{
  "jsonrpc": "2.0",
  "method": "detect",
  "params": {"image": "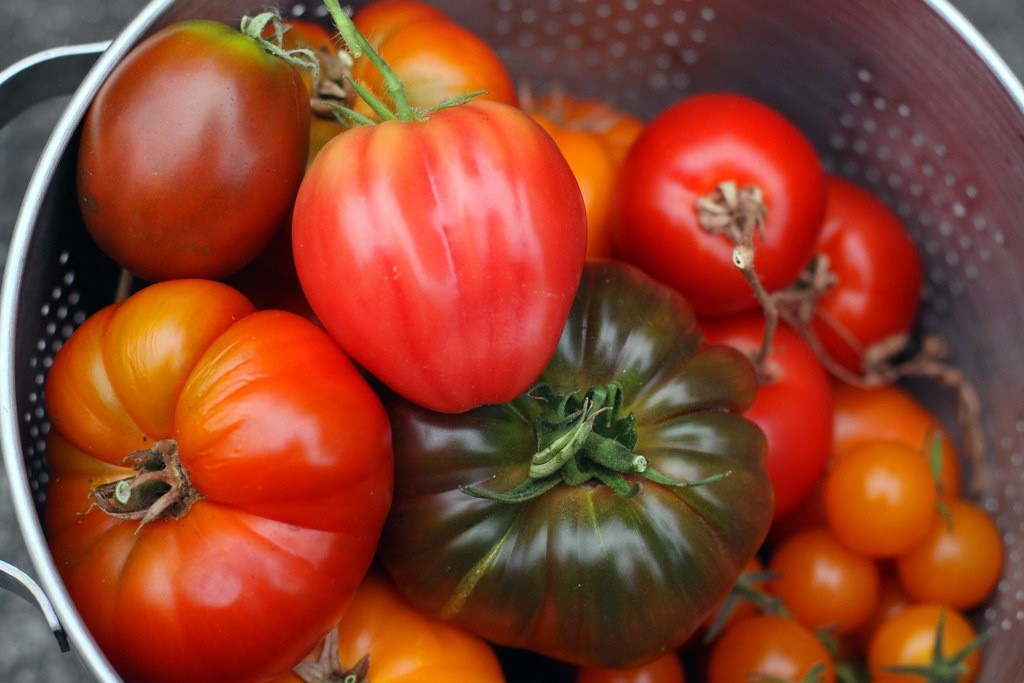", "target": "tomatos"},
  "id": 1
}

[40,2,1007,683]
